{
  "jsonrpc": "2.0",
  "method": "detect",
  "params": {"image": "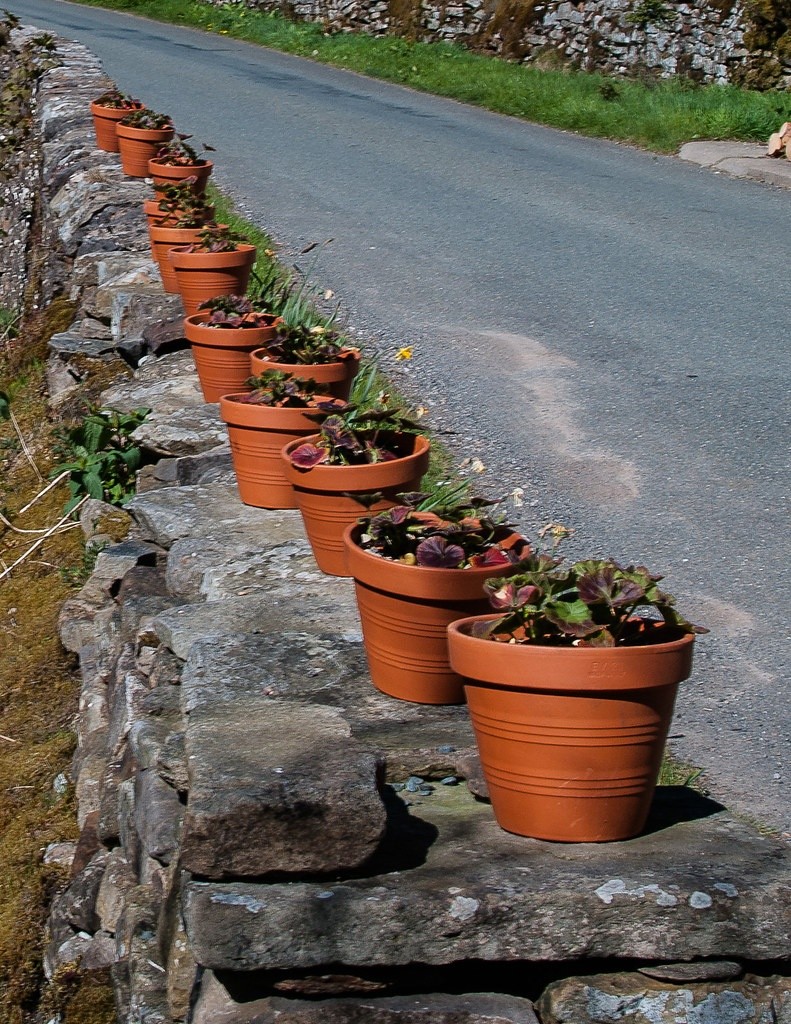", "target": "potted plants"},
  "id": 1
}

[343,489,531,706]
[282,390,433,577]
[116,107,177,178]
[90,83,146,151]
[447,556,696,844]
[167,226,257,316]
[145,174,217,260]
[150,198,230,295]
[148,133,213,200]
[249,320,360,403]
[219,368,347,508]
[185,294,286,403]
